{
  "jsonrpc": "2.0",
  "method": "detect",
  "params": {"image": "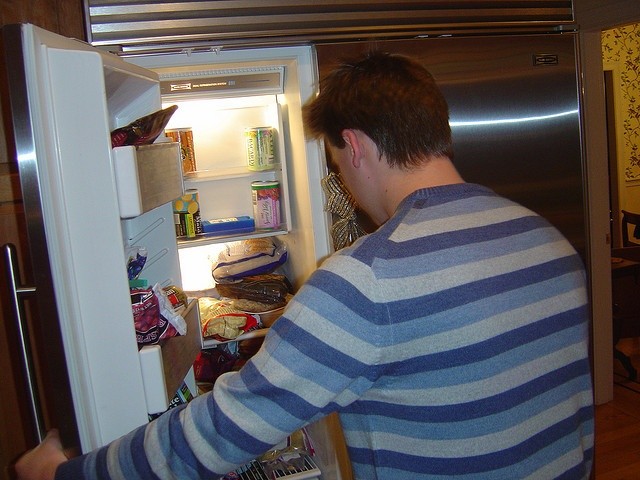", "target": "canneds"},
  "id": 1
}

[245,125,275,171]
[165,127,197,179]
[172,189,203,239]
[251,180,281,232]
